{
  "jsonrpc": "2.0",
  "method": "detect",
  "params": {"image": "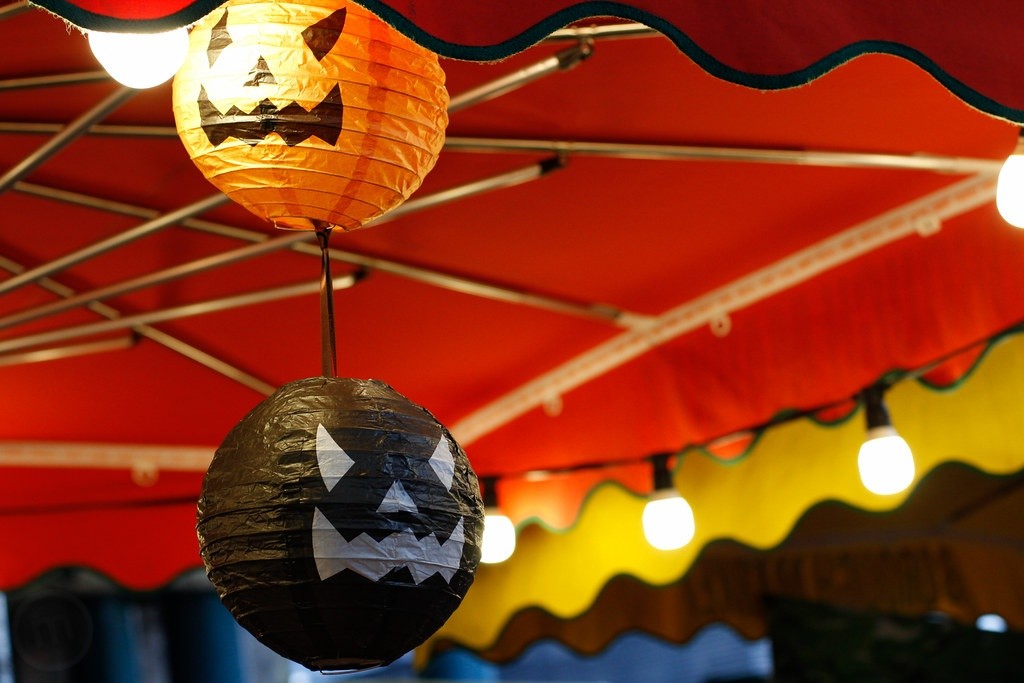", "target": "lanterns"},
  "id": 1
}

[172,0,453,234]
[197,375,483,671]
